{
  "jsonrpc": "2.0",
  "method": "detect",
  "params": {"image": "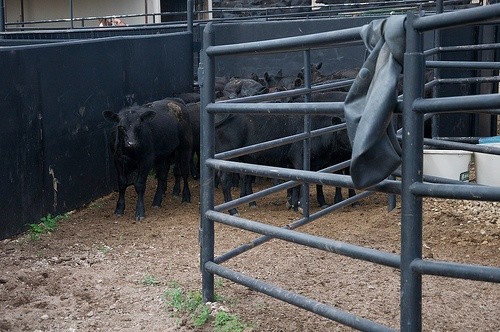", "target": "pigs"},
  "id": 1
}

[100,61,361,220]
